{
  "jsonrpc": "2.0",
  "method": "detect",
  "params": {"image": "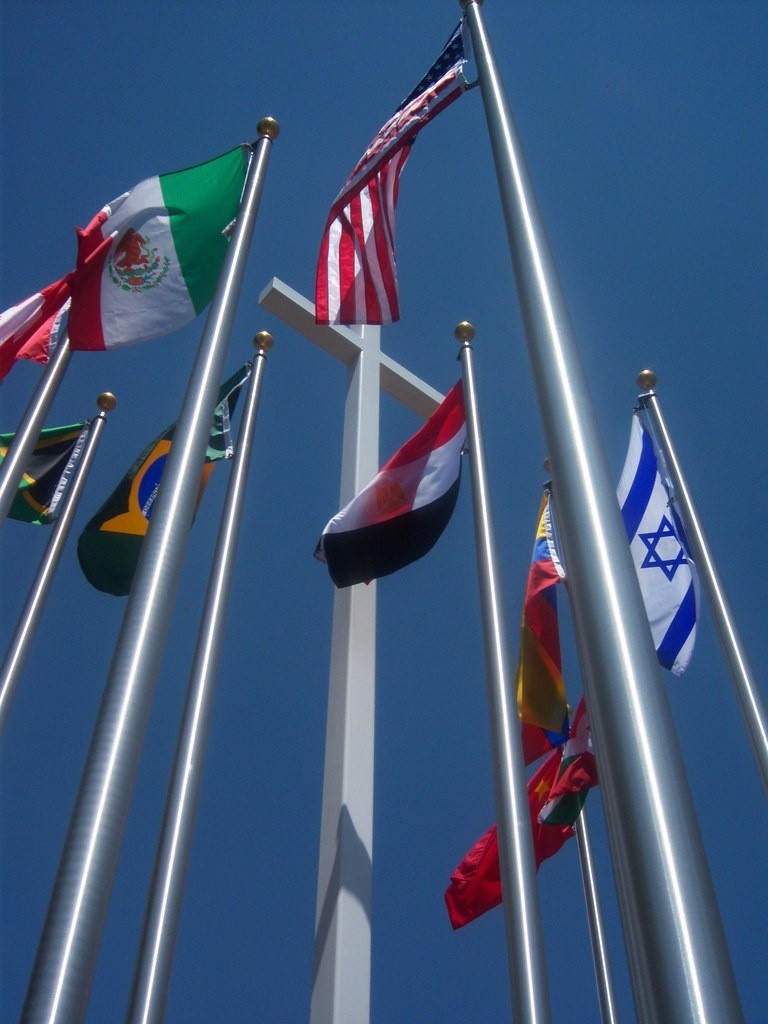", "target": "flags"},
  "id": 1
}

[445,695,598,935]
[2,274,83,382]
[2,422,96,526]
[65,134,256,355]
[74,357,254,598]
[614,407,706,677]
[510,490,571,767]
[317,370,468,589]
[299,13,477,330]
